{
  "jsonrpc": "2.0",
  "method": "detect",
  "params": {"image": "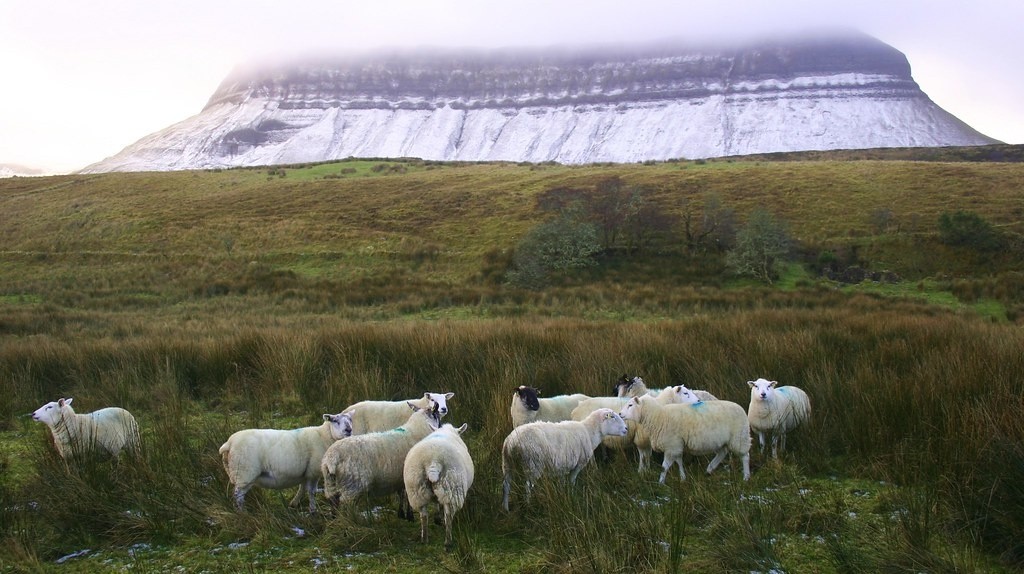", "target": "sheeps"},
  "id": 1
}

[321,401,441,520]
[217,409,357,514]
[32,397,140,466]
[340,392,456,432]
[403,423,475,547]
[502,408,629,514]
[747,378,812,459]
[510,386,590,429]
[571,372,751,485]
